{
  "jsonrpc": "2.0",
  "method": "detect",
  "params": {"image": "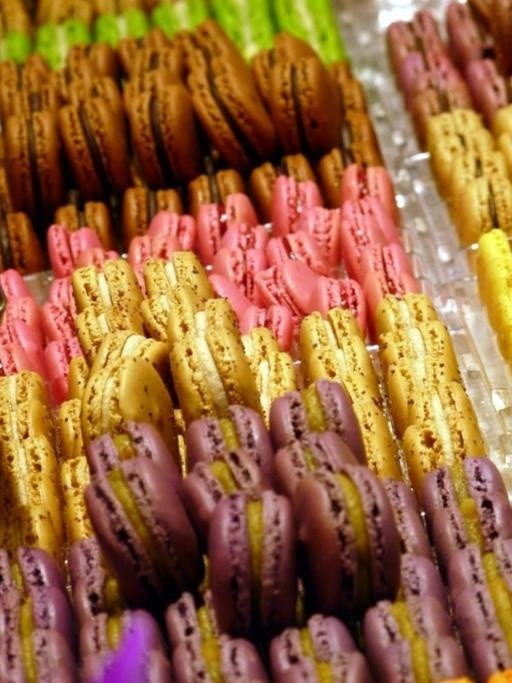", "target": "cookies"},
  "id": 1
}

[0,0,512,683]
